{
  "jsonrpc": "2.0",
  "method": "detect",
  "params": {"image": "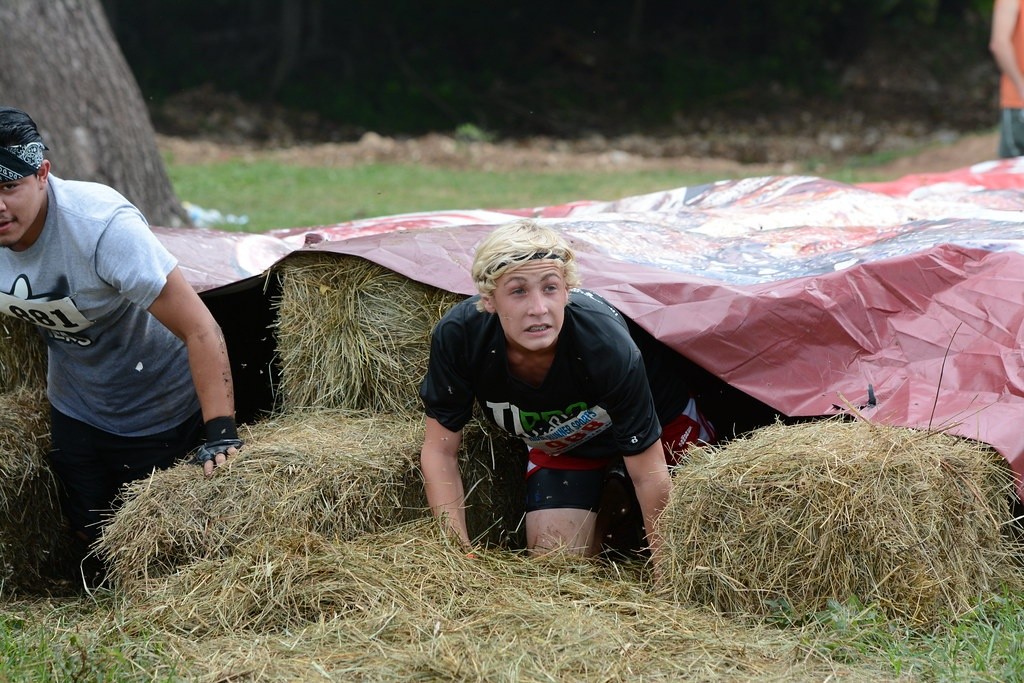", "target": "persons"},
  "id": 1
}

[0,109,242,590]
[988,0,1024,157]
[416,223,752,602]
